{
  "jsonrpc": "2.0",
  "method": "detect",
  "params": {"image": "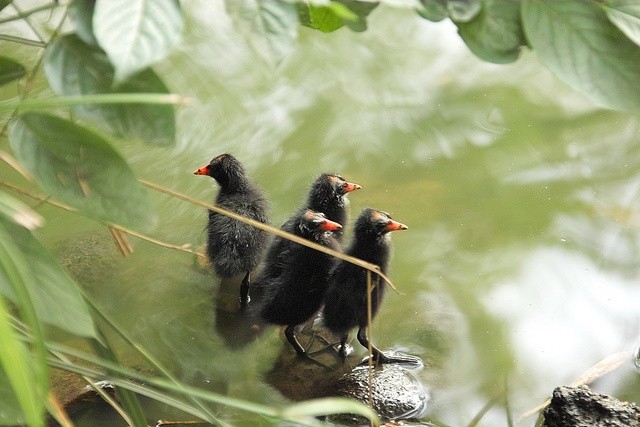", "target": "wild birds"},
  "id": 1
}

[302,174,362,245]
[192,154,273,306]
[254,208,343,370]
[321,209,421,370]
